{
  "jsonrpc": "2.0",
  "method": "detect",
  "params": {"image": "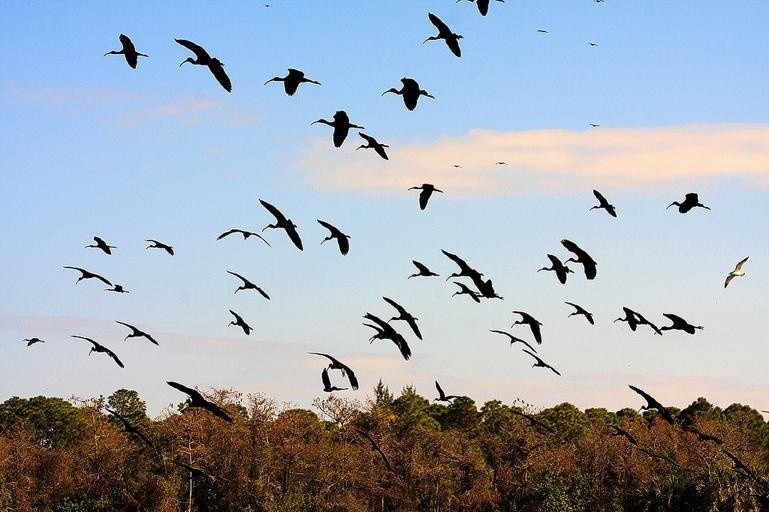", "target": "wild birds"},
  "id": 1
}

[175,39,231,93]
[26,336,40,345]
[62,183,764,496]
[106,34,147,69]
[266,67,433,159]
[427,12,463,57]
[469,0,501,17]
